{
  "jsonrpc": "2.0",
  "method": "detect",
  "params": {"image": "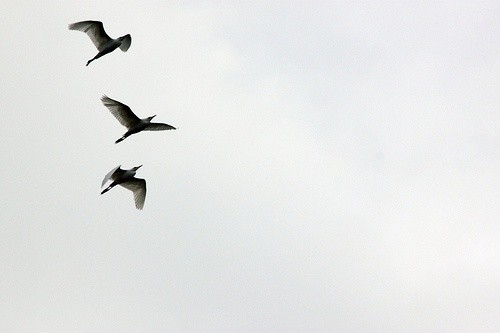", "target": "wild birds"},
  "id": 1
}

[68,20,132,67]
[100,165,146,210]
[101,94,176,145]
